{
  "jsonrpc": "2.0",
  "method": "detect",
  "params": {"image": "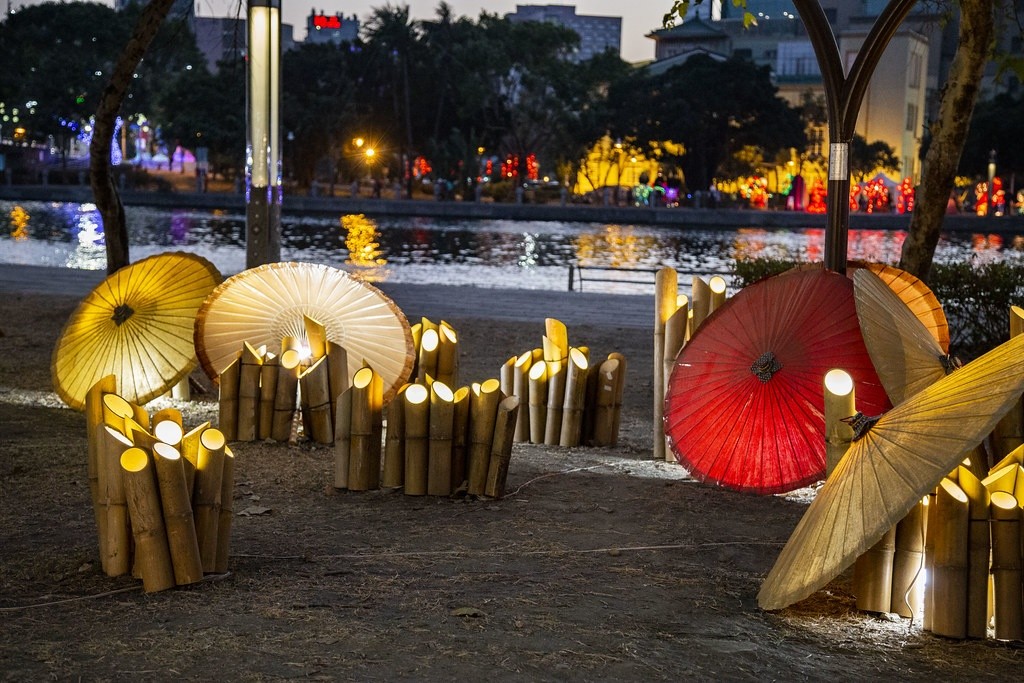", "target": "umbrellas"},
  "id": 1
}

[192,262,417,414]
[754,331,1024,611]
[854,264,959,407]
[50,251,223,411]
[662,266,893,495]
